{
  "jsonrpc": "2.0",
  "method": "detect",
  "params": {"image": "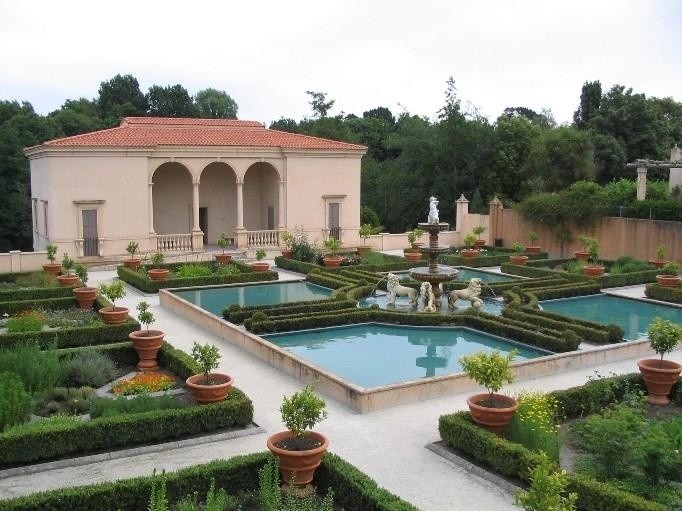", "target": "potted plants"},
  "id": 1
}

[583,240,605,278]
[265,378,330,498]
[73,263,97,311]
[129,301,165,372]
[355,224,372,252]
[472,224,487,249]
[123,241,141,271]
[57,253,79,288]
[636,317,682,406]
[42,245,62,277]
[649,244,668,269]
[575,235,595,262]
[280,232,297,259]
[510,242,528,265]
[214,231,232,267]
[655,262,680,288]
[148,251,170,281]
[323,237,344,267]
[185,340,234,404]
[97,281,129,325]
[410,228,425,250]
[459,348,521,433]
[525,229,541,252]
[251,248,270,272]
[404,232,422,261]
[460,234,480,257]
